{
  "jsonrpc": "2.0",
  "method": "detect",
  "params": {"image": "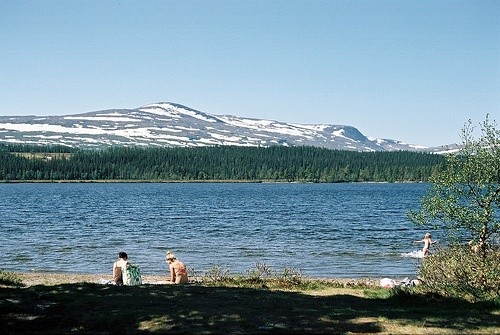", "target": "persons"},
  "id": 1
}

[113,252,128,285]
[165,251,188,283]
[414,233,437,255]
